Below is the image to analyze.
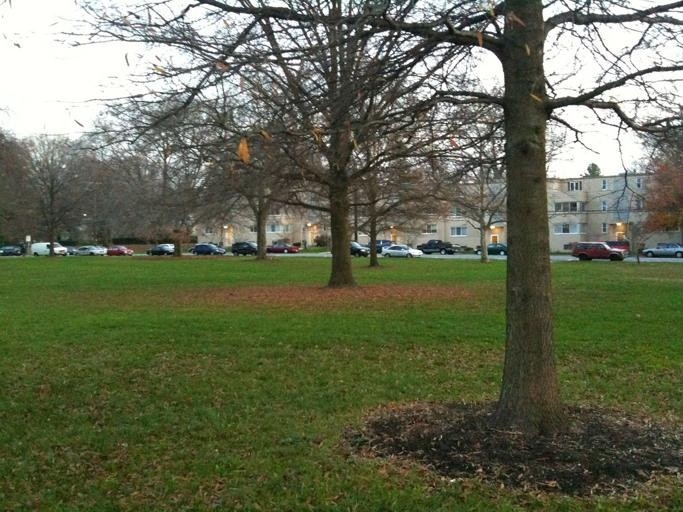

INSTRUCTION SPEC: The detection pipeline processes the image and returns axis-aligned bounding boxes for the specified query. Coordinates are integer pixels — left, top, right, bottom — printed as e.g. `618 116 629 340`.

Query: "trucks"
30 242 67 256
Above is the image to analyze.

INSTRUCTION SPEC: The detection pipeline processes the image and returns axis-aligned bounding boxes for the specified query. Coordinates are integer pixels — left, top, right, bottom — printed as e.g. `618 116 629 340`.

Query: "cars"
146 246 174 256
192 244 225 255
474 243 507 255
330 242 370 258
380 245 423 258
106 246 133 256
642 243 683 258
157 244 174 248
64 245 107 256
188 242 220 252
0 246 23 256
266 243 298 253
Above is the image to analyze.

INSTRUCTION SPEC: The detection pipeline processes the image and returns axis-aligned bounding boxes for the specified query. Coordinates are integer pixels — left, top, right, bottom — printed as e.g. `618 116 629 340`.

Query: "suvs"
231 242 257 256
572 242 625 261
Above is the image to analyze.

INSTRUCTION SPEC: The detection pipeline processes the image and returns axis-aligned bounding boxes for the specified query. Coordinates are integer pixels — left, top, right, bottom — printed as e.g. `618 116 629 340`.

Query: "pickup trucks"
416 240 461 255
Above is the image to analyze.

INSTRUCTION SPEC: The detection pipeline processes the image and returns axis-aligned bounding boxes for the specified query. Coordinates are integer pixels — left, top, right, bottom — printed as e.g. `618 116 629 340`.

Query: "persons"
303 240 306 249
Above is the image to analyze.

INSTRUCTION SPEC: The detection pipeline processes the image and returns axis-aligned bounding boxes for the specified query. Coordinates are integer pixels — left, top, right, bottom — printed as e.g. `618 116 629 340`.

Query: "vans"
368 240 391 253
597 240 629 249
